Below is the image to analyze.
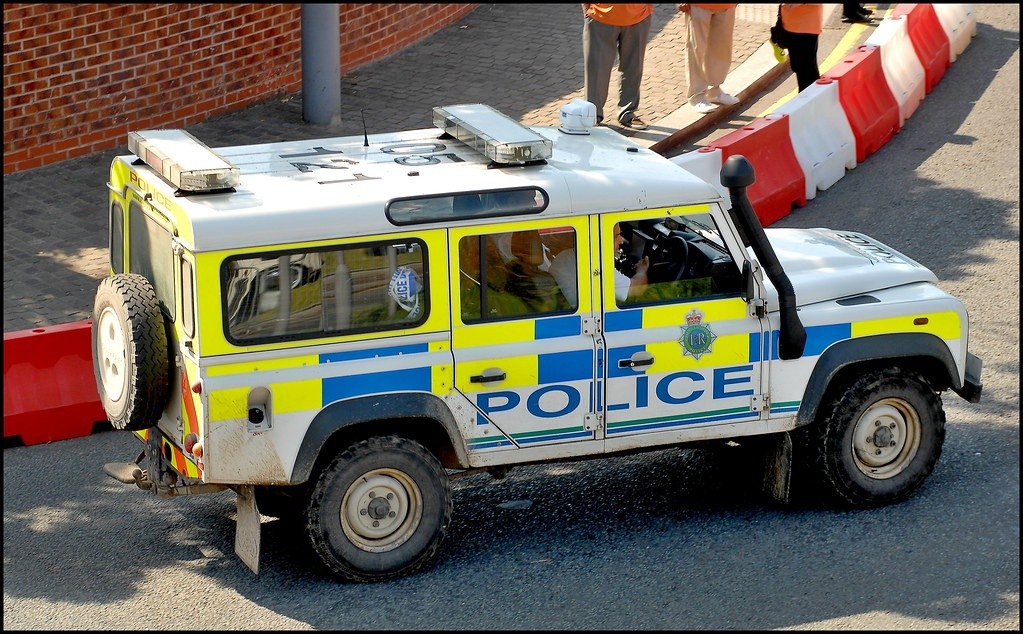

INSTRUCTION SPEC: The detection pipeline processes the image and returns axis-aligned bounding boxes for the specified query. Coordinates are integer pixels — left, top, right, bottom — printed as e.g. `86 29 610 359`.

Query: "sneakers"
769 37 787 64
707 91 740 105
622 117 646 130
692 97 715 114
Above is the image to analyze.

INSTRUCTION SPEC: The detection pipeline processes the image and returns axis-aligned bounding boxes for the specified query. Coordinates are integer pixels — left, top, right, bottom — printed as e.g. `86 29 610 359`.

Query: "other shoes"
842 15 874 23
856 6 873 15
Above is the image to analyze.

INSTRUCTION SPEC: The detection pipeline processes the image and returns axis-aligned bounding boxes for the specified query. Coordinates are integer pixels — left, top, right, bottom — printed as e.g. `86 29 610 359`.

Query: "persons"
774 2 821 93
548 221 653 309
579 1 656 127
677 2 737 115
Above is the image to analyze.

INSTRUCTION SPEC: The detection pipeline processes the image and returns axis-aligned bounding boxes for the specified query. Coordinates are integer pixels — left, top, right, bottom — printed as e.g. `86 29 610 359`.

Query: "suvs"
90 98 983 580
